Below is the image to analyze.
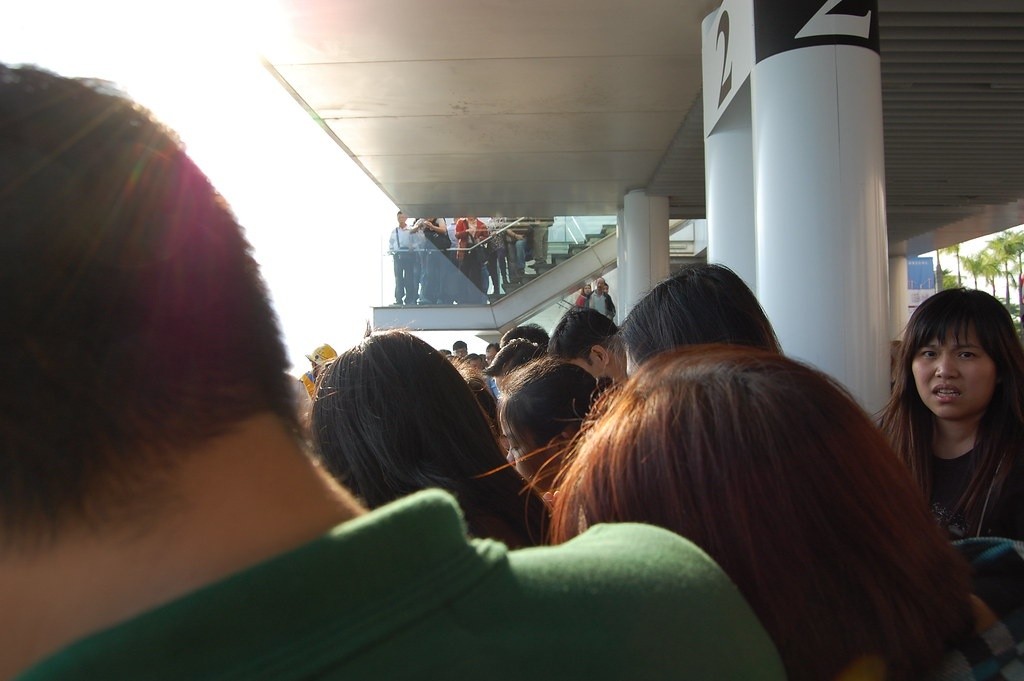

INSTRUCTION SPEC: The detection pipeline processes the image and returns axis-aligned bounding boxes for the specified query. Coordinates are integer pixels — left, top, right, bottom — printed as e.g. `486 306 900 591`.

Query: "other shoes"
528 261 546 268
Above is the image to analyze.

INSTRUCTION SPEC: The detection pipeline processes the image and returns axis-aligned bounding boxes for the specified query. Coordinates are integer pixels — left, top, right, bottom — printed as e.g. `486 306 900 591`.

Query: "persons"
0 74 1024 681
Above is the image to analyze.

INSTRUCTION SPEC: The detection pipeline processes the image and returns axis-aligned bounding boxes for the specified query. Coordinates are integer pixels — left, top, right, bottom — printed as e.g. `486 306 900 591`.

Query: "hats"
305 343 338 367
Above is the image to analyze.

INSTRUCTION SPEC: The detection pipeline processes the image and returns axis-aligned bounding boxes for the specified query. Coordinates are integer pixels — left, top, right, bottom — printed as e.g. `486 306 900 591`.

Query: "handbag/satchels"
464 233 488 269
425 217 451 250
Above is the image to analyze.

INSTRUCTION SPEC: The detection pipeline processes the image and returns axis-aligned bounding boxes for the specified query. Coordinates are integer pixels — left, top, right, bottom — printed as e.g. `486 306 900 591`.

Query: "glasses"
453 350 467 353
